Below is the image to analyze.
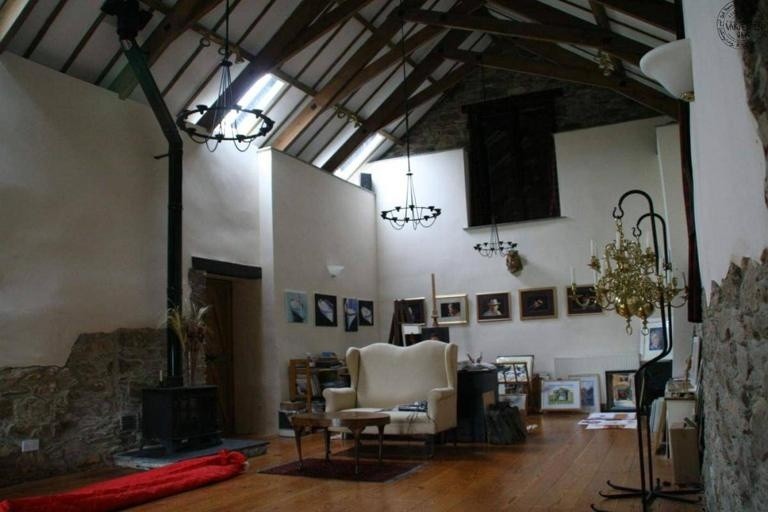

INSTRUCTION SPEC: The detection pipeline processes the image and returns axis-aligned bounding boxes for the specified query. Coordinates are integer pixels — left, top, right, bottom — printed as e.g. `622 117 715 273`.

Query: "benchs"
322 340 459 459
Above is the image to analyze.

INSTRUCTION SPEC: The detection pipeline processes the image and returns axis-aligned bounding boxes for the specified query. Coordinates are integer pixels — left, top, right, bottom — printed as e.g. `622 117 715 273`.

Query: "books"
295 374 321 398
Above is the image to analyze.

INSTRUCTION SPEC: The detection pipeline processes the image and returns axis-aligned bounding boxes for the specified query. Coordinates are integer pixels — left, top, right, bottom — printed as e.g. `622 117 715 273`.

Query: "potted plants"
158 296 217 386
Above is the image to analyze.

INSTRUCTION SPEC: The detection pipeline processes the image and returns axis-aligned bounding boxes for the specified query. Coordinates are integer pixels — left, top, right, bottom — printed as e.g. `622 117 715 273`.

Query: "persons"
446 304 460 316
482 299 502 316
648 329 663 350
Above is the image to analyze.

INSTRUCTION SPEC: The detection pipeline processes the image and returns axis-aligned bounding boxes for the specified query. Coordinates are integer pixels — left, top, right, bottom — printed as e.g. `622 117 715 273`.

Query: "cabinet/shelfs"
140 385 224 456
444 360 507 443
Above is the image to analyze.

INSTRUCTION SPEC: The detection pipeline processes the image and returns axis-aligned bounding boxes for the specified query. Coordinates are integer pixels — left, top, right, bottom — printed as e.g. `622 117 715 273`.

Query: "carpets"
257 457 422 485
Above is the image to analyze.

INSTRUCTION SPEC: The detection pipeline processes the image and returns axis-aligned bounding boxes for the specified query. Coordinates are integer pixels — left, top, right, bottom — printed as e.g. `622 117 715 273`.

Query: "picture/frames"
393 297 426 326
565 284 605 315
476 292 512 322
517 286 558 321
494 317 670 415
434 294 469 326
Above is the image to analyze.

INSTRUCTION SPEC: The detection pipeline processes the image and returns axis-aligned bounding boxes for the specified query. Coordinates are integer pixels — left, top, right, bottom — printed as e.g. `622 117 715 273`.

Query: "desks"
291 410 390 475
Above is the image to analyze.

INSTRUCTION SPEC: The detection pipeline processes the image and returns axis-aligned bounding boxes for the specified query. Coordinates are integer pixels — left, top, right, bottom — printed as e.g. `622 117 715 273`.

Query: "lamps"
326 265 345 278
585 187 688 336
173 0 275 152
472 66 518 259
639 38 693 103
378 0 442 231
